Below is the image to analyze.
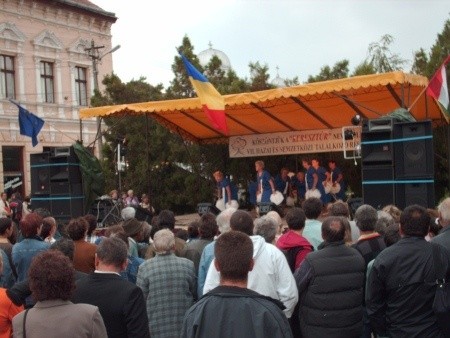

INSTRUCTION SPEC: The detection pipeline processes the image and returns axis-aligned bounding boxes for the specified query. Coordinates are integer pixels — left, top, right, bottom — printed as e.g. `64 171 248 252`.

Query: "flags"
426 58 450 117
181 54 227 134
19 106 44 147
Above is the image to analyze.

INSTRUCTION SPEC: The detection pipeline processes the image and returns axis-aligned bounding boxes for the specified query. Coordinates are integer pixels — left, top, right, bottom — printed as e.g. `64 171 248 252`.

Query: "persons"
239 197 314 272
182 230 294 338
352 204 387 338
301 197 325 251
291 216 365 338
427 197 450 248
328 201 401 276
199 211 234 299
150 210 218 261
12 248 108 338
137 228 198 338
364 205 450 338
0 255 24 338
213 157 343 212
72 237 151 338
202 212 299 318
0 189 155 308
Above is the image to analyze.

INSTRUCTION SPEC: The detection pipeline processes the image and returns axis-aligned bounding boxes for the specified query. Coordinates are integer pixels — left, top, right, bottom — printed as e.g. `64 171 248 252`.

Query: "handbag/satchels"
284 246 304 273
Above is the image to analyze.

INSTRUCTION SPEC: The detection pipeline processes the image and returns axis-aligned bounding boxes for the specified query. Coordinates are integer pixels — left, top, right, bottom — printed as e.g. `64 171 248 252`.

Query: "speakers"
29 146 95 231
361 117 435 211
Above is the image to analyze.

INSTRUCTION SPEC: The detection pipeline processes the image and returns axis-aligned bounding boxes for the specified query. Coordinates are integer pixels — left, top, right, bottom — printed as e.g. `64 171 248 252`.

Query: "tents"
79 70 450 207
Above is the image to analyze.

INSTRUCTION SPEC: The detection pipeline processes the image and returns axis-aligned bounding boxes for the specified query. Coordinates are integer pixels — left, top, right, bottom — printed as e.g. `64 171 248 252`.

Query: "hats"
123 218 143 236
